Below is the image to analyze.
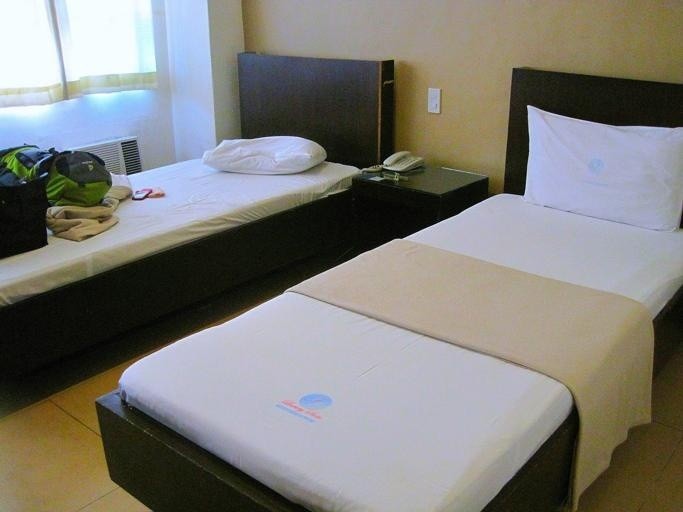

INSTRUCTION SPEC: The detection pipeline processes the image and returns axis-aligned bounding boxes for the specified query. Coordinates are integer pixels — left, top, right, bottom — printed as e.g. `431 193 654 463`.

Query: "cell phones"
132 188 153 200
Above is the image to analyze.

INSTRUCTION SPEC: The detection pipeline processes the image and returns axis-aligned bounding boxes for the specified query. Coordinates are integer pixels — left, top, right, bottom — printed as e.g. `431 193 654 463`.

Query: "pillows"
202 135 326 176
525 105 683 232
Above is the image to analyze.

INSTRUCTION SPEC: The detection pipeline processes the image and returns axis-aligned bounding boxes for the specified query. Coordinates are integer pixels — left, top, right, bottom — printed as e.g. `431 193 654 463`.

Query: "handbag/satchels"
0 162 48 259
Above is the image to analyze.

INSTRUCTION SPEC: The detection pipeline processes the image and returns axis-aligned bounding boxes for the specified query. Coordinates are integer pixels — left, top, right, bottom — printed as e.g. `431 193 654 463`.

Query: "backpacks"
0 143 112 206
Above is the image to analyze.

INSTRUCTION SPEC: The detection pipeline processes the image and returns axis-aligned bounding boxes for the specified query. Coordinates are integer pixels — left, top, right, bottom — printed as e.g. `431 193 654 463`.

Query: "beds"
94 193 683 512
2 156 379 417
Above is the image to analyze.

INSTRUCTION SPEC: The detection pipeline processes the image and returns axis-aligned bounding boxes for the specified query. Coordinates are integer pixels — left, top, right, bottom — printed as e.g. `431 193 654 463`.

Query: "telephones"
382 151 424 174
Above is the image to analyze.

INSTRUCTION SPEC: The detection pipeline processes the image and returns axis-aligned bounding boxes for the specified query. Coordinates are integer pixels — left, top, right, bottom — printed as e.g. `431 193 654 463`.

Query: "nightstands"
353 164 491 248
